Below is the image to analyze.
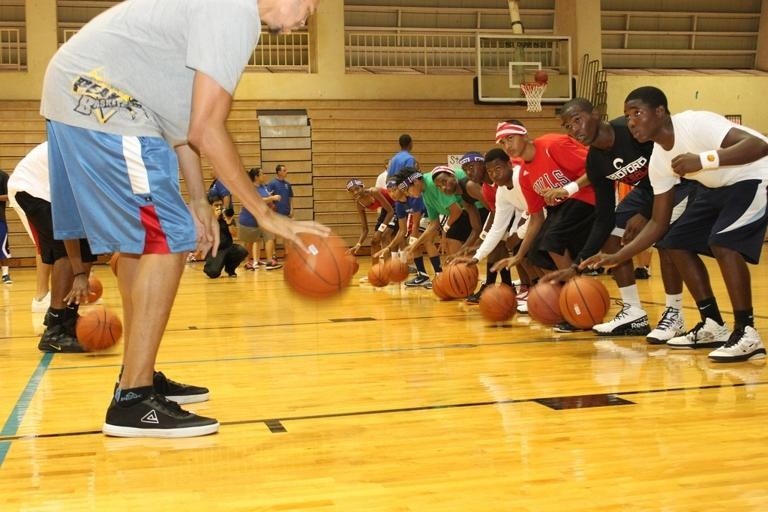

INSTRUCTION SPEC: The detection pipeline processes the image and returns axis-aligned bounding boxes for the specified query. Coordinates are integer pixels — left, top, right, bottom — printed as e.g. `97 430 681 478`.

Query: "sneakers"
1 274 12 284
265 261 282 270
103 395 219 439
646 306 686 344
245 262 254 270
517 301 528 312
553 322 584 332
43 310 80 325
225 269 237 277
359 275 369 283
582 268 598 276
114 372 210 405
253 261 259 269
592 301 651 336
32 292 51 312
667 318 730 348
38 325 82 352
515 287 528 300
259 261 265 265
708 325 766 361
424 279 432 289
634 266 651 279
404 273 430 286
464 284 485 305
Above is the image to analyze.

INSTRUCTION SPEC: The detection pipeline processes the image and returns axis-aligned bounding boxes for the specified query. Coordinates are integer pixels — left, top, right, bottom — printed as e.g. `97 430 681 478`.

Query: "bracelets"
73 271 86 277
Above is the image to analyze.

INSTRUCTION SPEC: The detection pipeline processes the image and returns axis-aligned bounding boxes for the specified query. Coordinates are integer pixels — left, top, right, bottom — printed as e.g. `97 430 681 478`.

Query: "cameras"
217 208 234 221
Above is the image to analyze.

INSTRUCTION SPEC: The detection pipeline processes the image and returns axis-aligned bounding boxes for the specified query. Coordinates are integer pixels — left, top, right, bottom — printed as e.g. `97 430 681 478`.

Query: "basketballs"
385 259 409 282
432 274 454 299
479 284 517 322
351 255 360 274
527 280 565 324
286 227 354 298
369 263 390 286
559 276 610 329
535 70 548 83
80 275 103 305
442 262 478 298
76 307 122 351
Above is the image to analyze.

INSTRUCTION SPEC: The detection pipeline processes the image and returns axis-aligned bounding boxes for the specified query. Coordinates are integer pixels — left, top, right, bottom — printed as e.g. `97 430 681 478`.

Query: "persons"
203 194 249 277
14 196 52 310
238 168 282 270
0 171 13 283
35 0 316 439
267 165 294 258
209 168 233 213
6 138 102 352
347 87 766 363
